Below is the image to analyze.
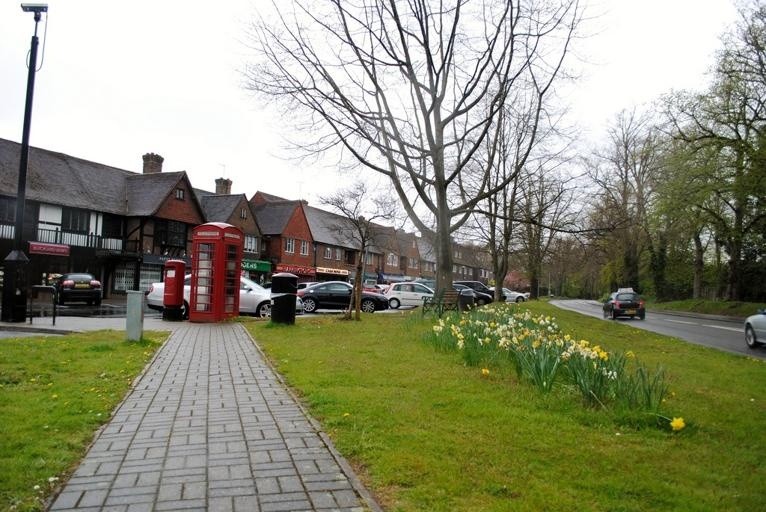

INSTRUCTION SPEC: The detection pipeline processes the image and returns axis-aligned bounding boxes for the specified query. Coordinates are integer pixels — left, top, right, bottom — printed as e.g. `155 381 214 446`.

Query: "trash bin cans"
271 272 299 323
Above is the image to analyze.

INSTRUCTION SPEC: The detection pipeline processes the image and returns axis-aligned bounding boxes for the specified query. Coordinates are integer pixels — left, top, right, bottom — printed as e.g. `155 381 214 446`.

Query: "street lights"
11 2 47 251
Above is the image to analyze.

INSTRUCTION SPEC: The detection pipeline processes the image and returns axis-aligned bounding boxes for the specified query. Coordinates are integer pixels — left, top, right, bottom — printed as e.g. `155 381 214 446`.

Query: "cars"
146 270 304 319
603 287 646 320
298 280 528 314
52 273 103 307
744 308 766 348
